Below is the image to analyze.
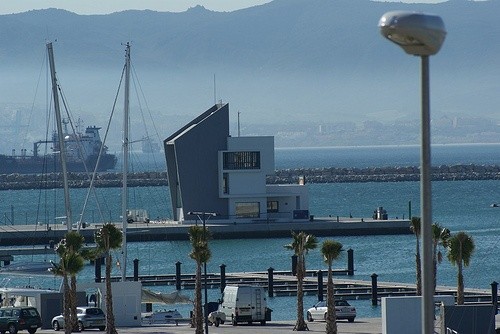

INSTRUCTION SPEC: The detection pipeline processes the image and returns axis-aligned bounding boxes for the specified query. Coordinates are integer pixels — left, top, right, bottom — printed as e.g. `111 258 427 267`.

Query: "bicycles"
154 216 167 224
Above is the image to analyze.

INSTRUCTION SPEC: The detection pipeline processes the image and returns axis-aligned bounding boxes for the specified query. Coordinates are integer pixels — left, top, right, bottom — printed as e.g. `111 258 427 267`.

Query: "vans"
218 284 268 327
127 209 150 223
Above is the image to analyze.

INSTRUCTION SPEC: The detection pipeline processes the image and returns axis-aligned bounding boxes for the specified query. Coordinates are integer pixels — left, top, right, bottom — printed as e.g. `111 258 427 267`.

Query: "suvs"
0 306 44 334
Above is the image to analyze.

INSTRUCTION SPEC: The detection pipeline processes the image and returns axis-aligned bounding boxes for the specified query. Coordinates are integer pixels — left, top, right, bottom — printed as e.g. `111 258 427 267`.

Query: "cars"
307 300 357 322
51 307 107 332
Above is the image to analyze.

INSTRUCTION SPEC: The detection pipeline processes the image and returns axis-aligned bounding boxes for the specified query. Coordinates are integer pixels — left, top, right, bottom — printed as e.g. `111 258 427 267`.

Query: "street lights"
378 10 448 334
187 210 221 334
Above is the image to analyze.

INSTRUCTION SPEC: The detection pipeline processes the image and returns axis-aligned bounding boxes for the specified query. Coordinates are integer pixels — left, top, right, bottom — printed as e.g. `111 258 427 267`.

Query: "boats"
0 117 117 173
